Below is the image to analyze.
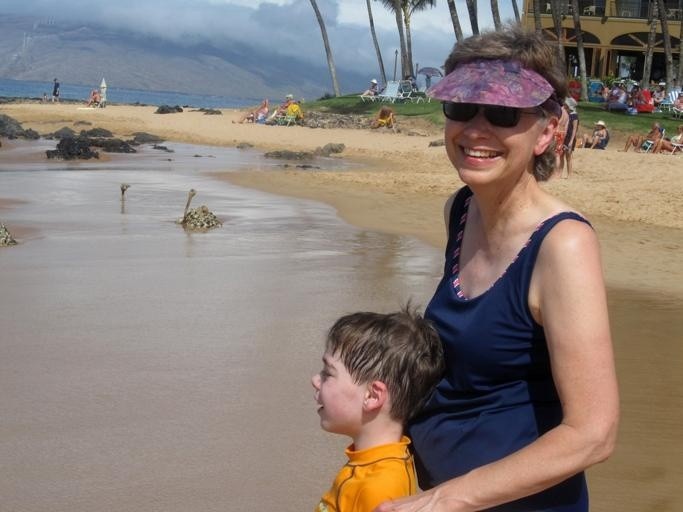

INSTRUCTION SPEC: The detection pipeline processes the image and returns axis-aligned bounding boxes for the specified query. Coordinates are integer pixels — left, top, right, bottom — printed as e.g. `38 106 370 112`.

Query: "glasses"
438 97 548 129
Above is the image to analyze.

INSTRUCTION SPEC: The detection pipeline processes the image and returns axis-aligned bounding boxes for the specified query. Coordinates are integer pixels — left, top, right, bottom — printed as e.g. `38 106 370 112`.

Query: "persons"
361 79 378 97
85 90 100 107
307 303 446 511
374 106 395 128
375 28 624 512
264 94 297 125
51 77 59 104
558 77 683 156
556 96 579 180
231 98 268 127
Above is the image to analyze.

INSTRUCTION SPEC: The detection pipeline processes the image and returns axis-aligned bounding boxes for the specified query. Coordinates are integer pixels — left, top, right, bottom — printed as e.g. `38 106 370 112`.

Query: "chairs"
591 129 609 149
359 79 432 104
637 128 683 155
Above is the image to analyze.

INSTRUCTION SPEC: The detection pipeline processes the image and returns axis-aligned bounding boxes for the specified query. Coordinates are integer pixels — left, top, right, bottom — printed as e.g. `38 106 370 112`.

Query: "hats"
594 120 606 128
370 78 378 85
285 94 294 100
564 96 577 111
424 58 561 111
